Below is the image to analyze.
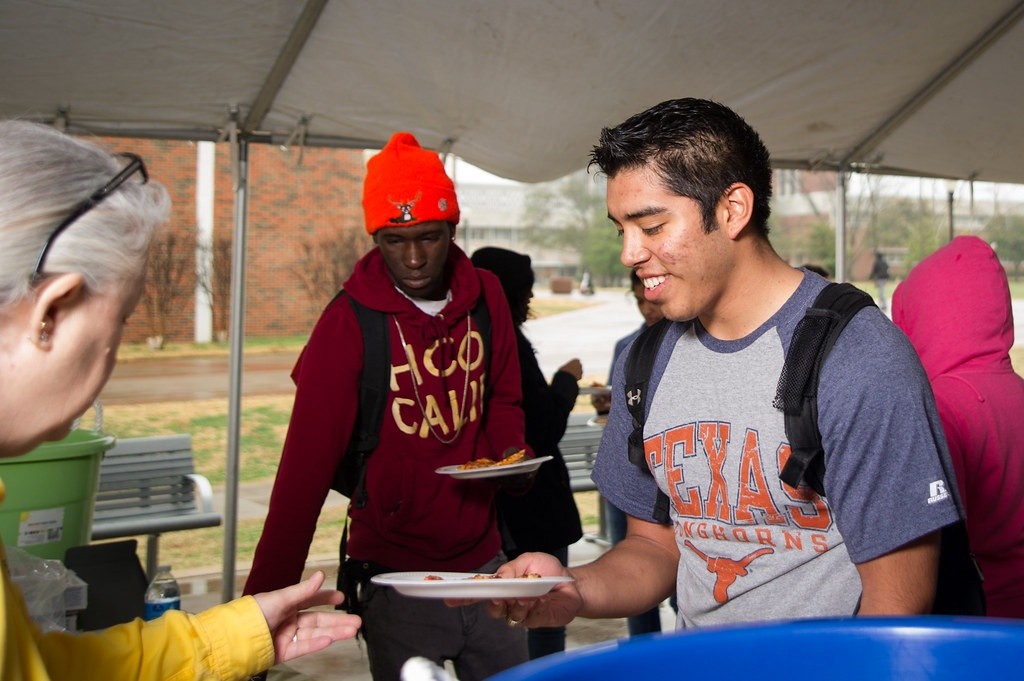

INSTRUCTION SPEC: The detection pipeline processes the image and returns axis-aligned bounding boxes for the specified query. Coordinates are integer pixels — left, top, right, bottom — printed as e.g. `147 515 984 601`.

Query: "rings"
292 627 300 644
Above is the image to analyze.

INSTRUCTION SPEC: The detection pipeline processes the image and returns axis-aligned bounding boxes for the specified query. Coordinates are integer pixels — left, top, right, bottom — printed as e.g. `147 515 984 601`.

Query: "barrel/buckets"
0 428 116 564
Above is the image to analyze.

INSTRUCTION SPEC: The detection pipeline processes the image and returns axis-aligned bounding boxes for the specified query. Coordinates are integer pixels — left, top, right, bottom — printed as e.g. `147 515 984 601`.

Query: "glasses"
29 151 150 307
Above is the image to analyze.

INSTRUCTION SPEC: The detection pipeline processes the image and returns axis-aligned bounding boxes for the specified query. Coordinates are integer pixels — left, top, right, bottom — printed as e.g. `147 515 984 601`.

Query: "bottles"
145 564 180 621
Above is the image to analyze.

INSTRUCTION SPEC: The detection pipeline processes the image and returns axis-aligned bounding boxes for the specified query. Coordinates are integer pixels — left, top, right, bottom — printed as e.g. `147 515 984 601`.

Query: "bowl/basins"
482 615 1024 681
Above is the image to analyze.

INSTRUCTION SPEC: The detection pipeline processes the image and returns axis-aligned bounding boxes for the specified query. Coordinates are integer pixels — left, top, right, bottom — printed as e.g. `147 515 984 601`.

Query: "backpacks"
931 519 988 616
334 557 367 642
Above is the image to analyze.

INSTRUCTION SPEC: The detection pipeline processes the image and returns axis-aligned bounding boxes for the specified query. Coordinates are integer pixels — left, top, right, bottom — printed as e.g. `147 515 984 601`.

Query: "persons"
240 98 1024 681
0 119 362 681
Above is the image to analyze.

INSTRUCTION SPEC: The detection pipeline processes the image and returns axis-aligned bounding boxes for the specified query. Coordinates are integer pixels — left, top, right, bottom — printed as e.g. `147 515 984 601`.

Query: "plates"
587 416 610 427
371 572 573 598
434 455 554 479
579 385 612 394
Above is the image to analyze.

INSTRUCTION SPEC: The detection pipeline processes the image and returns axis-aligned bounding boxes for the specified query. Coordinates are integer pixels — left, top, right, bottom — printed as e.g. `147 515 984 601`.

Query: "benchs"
91 433 222 586
557 412 608 542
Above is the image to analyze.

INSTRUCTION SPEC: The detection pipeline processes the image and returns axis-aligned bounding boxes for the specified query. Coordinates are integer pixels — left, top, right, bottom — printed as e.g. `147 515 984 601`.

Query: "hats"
361 131 461 235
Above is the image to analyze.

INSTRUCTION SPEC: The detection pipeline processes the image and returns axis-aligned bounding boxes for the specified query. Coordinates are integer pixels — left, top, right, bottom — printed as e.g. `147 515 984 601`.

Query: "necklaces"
390 312 472 443
